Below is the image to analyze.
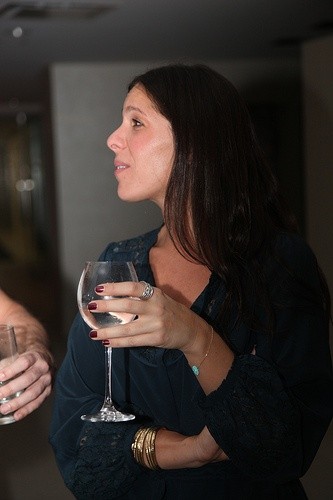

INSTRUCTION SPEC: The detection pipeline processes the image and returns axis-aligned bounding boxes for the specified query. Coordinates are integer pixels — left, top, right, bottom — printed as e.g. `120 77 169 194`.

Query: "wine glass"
77 262 139 422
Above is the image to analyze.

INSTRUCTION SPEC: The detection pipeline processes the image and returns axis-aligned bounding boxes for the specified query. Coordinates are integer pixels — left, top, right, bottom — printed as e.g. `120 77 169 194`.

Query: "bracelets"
132 428 162 472
191 327 214 375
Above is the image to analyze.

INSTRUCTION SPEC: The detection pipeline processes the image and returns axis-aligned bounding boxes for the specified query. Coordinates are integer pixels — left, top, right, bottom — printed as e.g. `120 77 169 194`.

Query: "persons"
50 62 333 500
0 286 53 426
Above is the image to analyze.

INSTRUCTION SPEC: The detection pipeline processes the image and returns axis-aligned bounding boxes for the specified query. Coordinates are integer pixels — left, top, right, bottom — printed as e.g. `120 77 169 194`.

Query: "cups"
0 324 25 425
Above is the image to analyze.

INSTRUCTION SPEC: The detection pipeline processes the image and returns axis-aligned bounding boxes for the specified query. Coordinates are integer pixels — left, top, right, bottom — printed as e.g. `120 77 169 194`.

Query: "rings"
141 282 154 301
